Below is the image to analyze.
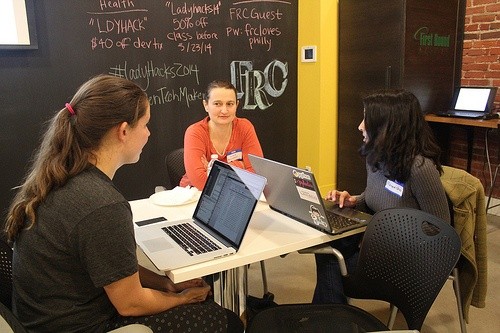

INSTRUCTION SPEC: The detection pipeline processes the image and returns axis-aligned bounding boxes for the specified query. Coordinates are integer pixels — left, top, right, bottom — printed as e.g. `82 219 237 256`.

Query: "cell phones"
135 217 167 227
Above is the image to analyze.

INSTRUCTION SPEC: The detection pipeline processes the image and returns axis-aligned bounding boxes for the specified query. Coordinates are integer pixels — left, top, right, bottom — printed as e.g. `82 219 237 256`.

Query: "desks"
425 114 500 174
128 189 367 333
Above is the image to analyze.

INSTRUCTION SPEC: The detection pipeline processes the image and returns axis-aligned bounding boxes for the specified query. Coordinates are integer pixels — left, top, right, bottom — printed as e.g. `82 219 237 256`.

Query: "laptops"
247 153 373 236
133 159 267 272
434 86 498 119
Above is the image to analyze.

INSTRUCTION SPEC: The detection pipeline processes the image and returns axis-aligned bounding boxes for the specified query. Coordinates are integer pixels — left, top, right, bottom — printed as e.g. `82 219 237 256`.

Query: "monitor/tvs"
0 0 38 50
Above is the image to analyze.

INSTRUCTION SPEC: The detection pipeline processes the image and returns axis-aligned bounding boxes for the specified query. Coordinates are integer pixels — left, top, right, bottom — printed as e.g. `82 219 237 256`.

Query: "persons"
5 74 245 333
179 80 264 282
310 89 451 305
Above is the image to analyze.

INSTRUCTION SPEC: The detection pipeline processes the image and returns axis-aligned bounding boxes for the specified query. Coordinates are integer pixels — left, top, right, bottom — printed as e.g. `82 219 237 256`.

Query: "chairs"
247 207 463 333
387 165 485 333
164 148 269 296
